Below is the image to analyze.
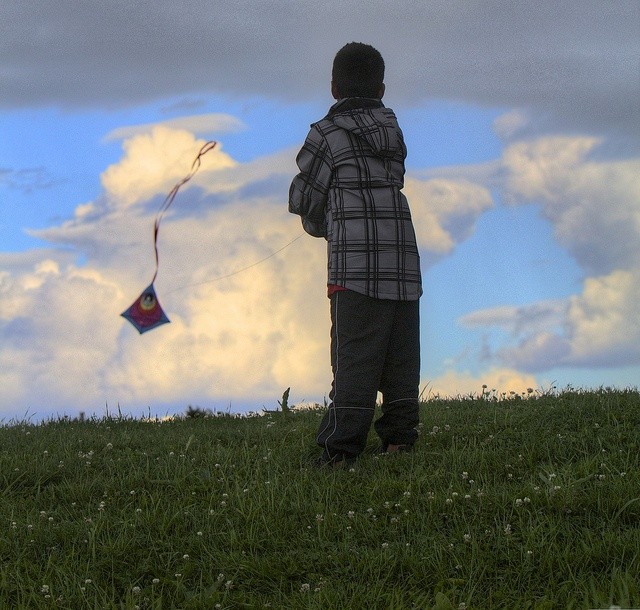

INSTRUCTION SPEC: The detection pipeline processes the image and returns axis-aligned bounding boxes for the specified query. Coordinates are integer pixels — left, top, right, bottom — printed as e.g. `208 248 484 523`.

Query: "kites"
116 140 217 337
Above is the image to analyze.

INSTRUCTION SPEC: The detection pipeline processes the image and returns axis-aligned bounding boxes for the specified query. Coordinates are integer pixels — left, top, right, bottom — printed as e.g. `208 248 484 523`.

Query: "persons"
289 41 422 461
